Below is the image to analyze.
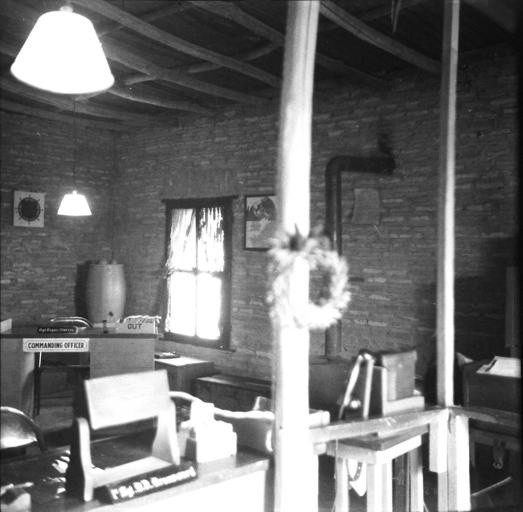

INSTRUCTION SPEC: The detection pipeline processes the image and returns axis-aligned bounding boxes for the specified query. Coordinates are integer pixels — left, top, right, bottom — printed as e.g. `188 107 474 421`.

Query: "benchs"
190 371 271 413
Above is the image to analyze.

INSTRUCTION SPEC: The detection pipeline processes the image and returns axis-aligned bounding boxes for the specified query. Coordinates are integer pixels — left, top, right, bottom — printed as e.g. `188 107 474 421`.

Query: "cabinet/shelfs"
154 354 213 407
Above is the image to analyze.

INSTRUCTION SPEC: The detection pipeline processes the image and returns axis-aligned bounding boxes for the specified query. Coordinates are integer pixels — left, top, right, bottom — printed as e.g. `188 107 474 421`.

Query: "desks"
1 423 269 509
1 322 157 420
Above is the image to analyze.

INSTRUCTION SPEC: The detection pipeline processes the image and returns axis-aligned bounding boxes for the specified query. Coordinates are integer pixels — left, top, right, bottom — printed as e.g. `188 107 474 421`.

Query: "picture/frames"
242 193 277 252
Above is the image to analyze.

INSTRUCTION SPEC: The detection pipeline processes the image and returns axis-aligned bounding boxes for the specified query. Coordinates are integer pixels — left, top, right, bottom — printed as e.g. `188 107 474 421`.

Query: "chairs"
31 316 94 420
322 347 428 511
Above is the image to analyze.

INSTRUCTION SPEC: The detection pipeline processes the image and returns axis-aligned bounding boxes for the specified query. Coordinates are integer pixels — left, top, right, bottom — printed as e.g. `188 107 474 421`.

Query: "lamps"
8 3 116 218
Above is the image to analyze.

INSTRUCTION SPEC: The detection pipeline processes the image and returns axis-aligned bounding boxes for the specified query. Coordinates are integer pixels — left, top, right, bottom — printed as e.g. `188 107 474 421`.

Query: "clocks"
11 189 48 231
